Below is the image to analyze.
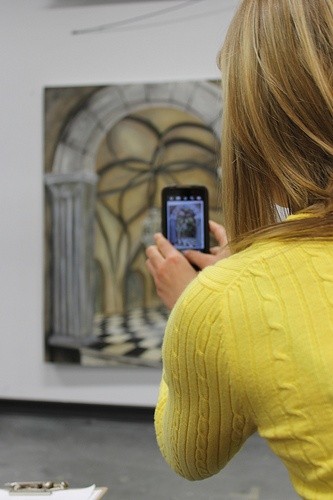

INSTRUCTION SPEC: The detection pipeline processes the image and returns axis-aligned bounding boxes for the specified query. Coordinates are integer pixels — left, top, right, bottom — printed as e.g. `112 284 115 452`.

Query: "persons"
136 1 333 500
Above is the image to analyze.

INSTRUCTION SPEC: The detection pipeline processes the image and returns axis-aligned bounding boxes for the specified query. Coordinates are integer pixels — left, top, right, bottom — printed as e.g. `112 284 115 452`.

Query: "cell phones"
161 185 210 271
